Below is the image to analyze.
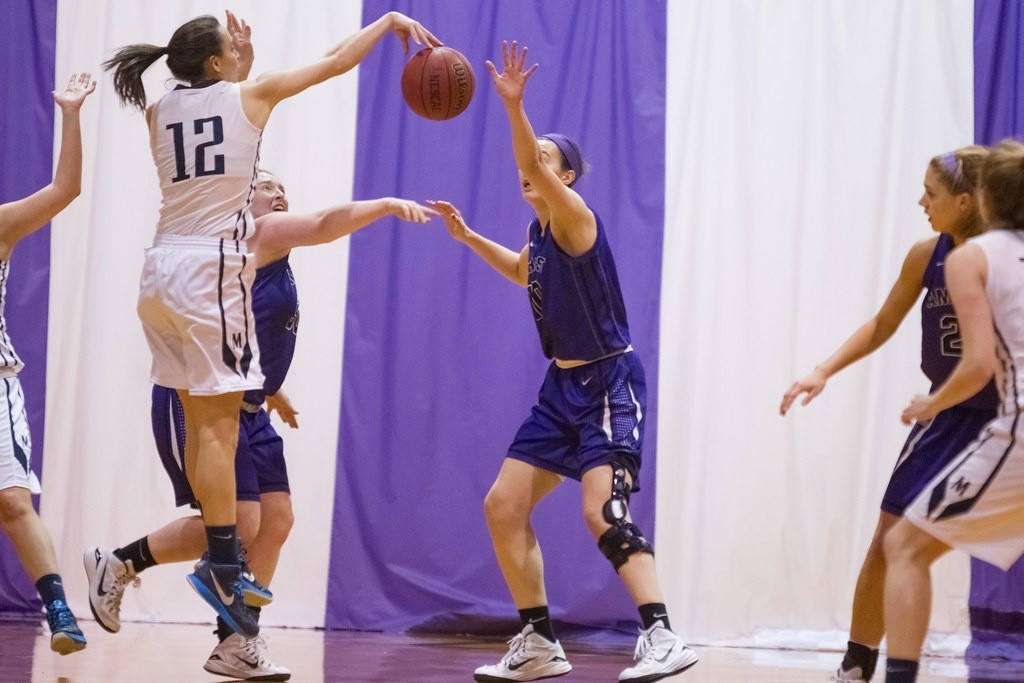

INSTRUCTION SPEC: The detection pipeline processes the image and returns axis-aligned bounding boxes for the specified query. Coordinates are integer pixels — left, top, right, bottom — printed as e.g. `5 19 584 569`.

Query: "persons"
424 35 699 683
778 136 1024 683
80 8 440 683
0 70 98 656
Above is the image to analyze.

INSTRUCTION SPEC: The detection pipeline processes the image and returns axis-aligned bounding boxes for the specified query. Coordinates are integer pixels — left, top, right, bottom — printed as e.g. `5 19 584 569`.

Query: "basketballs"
399 45 477 123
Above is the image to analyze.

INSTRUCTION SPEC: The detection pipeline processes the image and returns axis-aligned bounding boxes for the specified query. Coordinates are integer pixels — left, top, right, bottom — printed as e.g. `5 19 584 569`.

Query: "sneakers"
828 660 866 683
203 632 291 681
618 620 700 682
474 623 572 682
83 546 141 633
194 537 273 608
186 561 260 638
45 600 88 656
